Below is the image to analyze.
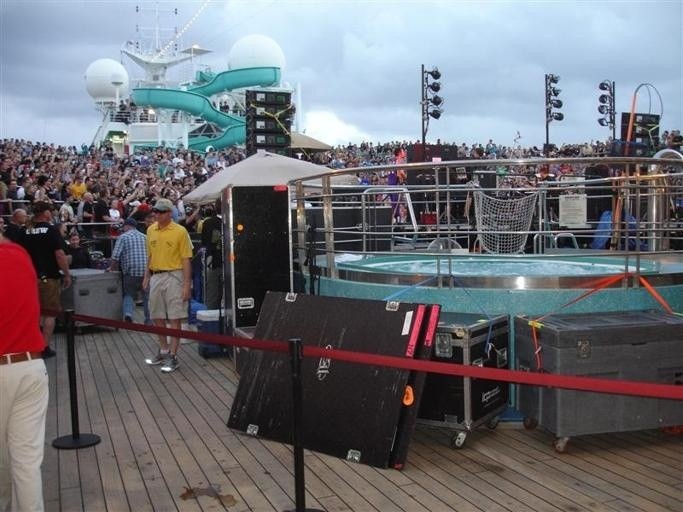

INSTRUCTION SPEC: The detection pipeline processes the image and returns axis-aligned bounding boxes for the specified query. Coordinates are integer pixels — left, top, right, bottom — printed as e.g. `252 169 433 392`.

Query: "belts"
0 352 43 363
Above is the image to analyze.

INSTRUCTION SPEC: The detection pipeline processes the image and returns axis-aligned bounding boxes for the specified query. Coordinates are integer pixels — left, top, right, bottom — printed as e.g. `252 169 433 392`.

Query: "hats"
33 201 55 212
122 218 138 226
152 198 173 212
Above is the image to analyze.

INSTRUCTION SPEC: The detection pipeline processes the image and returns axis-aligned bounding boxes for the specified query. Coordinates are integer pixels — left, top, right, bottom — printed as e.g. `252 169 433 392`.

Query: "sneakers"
145 347 171 364
42 345 55 358
160 355 180 372
124 311 133 322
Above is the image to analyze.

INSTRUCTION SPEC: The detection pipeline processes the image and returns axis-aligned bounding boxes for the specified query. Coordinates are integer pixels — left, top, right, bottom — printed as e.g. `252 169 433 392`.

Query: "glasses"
152 209 166 214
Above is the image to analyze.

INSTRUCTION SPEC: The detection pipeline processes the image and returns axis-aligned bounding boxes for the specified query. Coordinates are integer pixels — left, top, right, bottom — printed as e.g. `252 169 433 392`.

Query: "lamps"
599 83 614 127
427 69 441 118
546 73 564 122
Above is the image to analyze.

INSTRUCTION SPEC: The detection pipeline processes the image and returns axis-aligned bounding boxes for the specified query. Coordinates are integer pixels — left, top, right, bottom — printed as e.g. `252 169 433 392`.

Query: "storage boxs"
514 309 683 438
417 310 510 427
195 308 222 355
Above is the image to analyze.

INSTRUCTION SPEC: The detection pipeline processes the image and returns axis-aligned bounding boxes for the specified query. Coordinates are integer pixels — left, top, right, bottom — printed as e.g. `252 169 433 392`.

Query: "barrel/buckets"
195 309 219 357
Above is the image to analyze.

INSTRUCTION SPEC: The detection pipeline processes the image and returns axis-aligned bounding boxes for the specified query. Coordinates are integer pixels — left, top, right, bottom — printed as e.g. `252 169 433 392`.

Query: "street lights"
544 73 563 157
598 79 616 157
422 64 442 162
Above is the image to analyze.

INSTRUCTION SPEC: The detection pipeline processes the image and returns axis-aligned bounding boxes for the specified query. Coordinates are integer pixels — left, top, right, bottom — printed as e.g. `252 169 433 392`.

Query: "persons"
199 209 222 310
143 199 194 372
106 218 152 326
15 202 71 359
2 236 49 512
0 99 682 270
